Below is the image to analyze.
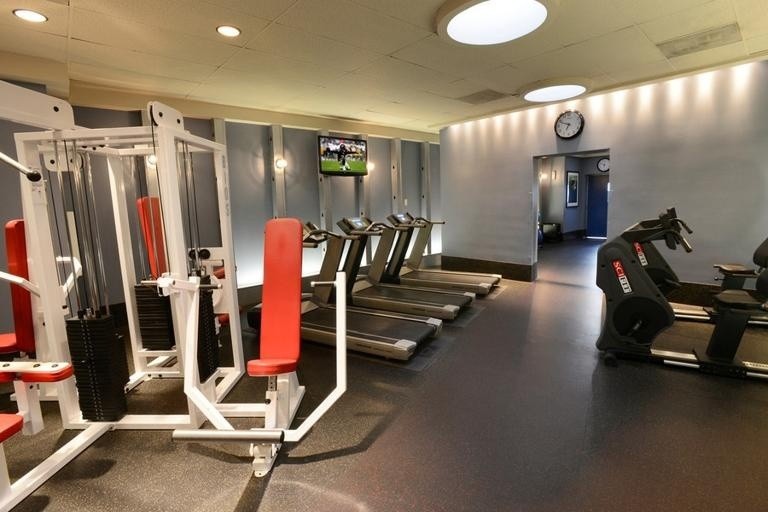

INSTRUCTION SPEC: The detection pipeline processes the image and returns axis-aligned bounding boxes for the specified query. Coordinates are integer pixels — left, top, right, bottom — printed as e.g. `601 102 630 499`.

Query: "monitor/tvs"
318 134 368 176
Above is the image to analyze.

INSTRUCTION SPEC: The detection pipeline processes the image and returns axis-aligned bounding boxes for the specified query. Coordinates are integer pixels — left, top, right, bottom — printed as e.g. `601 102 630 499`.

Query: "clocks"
554 110 584 140
597 158 609 172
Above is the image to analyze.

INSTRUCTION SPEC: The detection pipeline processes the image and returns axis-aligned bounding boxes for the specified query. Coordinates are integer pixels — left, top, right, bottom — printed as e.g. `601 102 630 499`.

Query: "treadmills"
247 223 442 360
384 213 502 295
329 218 475 322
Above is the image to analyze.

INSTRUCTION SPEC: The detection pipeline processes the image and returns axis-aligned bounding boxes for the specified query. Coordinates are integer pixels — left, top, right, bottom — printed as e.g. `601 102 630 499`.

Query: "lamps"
276 159 290 169
517 76 591 105
432 0 552 47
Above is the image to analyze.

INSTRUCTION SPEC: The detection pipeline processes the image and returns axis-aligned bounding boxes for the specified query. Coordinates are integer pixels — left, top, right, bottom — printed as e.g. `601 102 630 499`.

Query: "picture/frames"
566 171 579 208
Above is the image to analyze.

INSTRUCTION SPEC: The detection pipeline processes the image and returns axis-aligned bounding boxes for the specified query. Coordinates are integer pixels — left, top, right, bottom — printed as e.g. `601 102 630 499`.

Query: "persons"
570 179 577 192
320 137 365 172
